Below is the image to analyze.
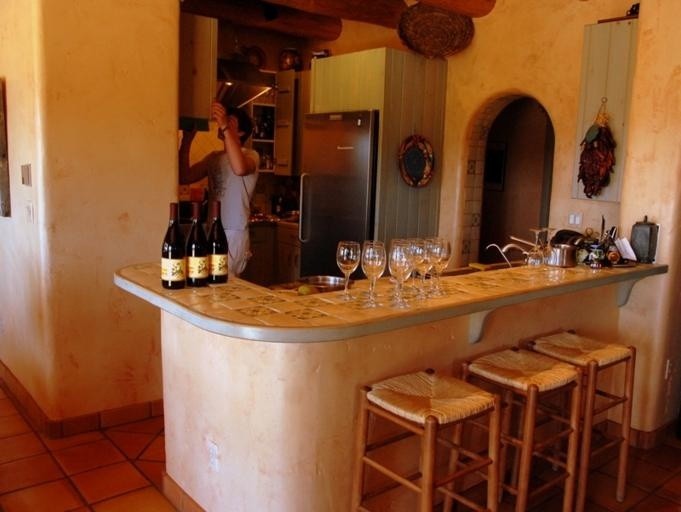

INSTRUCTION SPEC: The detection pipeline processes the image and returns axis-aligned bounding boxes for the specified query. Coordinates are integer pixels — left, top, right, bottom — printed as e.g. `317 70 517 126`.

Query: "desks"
113 247 668 511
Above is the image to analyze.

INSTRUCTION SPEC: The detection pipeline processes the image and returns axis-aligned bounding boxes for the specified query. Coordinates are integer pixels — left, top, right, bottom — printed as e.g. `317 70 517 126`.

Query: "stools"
511 329 638 511
351 369 503 511
443 342 587 512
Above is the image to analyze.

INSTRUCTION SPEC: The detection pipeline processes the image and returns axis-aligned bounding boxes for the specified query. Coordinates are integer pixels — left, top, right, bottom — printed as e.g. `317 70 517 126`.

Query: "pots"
510 234 580 269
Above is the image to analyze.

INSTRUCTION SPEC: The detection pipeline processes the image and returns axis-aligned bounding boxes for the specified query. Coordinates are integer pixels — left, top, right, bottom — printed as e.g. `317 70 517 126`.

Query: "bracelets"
219 125 231 134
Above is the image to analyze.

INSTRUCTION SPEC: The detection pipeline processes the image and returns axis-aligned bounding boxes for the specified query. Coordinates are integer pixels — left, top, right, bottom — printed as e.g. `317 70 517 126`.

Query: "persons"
177 103 261 278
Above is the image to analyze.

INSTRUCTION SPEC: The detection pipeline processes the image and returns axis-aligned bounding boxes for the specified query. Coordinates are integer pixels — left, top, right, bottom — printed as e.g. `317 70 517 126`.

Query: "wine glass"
526 225 558 270
335 237 453 308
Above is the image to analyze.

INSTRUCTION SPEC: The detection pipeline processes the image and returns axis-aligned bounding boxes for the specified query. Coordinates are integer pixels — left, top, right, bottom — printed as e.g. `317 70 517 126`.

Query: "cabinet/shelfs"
275 219 301 283
181 13 218 122
275 70 311 177
215 69 275 173
241 221 276 287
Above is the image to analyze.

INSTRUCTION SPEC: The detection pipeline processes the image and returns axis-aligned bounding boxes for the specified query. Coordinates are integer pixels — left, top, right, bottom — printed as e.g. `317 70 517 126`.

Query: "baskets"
397 0 476 58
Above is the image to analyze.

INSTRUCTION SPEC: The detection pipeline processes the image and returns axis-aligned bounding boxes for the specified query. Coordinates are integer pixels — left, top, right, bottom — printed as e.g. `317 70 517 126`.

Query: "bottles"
160 200 230 290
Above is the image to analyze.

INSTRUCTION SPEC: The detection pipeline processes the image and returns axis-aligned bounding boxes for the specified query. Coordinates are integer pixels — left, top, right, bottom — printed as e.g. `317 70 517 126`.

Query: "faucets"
502 243 527 256
485 244 513 268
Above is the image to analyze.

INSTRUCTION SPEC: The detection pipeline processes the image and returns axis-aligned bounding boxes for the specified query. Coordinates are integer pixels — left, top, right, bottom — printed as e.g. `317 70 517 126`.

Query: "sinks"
490 262 525 268
444 268 479 276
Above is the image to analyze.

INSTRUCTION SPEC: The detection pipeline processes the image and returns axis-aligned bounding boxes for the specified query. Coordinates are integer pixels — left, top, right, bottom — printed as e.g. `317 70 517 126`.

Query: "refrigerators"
298 112 379 277
